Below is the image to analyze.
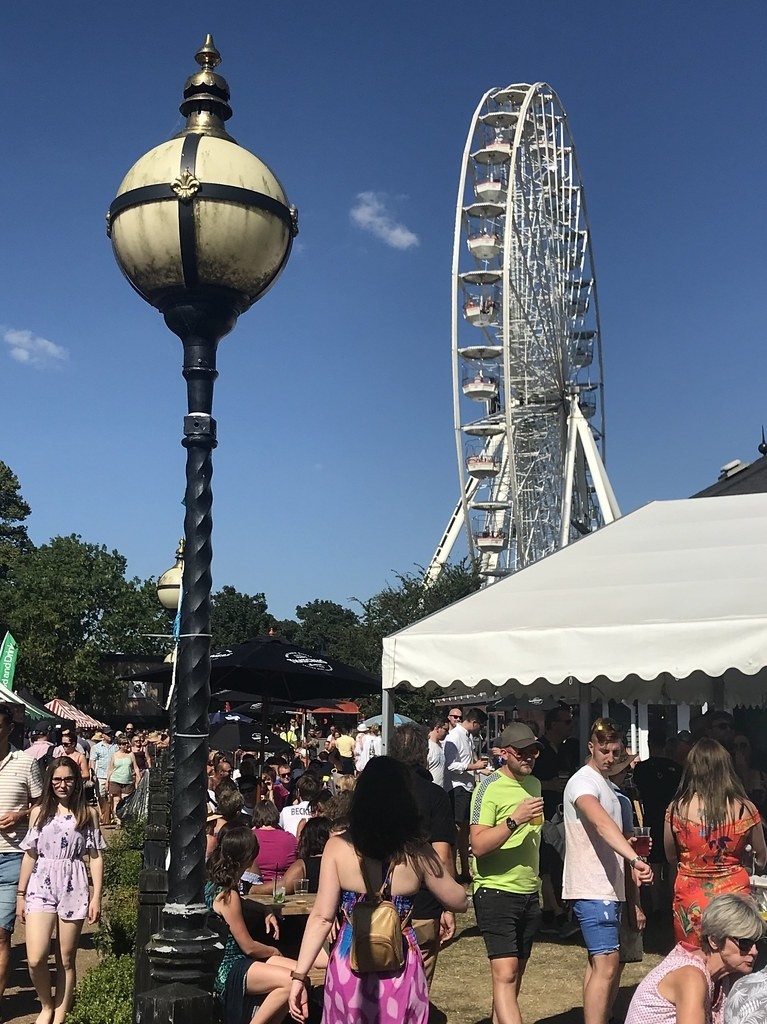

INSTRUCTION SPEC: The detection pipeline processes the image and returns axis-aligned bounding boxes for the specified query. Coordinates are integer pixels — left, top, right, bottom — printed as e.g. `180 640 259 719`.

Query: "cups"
481 757 489 767
273 877 287 904
633 826 651 856
527 796 542 826
293 878 309 905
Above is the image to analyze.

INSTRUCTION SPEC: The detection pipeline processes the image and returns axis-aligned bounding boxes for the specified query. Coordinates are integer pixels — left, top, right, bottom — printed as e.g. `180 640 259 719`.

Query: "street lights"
100 31 304 1024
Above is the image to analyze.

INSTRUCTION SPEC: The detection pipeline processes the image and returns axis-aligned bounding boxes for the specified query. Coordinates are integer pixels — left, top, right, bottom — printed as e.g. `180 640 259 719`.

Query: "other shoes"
98 816 122 830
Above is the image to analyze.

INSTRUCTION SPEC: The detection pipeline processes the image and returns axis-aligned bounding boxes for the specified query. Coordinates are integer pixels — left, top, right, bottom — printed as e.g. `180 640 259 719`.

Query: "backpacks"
329 830 414 976
37 744 56 782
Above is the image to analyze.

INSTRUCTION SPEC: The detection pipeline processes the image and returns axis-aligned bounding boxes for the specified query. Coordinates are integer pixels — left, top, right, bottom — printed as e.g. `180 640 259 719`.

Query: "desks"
239 892 317 917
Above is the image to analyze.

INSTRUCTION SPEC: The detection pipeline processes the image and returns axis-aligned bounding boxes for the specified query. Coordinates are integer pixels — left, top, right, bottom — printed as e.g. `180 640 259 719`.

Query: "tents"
1 681 111 730
379 490 767 761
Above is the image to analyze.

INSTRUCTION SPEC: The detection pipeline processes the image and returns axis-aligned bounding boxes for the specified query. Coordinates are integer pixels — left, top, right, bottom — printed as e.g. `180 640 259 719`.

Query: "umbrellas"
117 629 420 770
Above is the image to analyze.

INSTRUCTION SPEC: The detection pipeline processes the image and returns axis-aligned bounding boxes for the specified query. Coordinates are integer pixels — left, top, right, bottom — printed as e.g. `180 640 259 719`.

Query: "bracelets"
16 889 25 893
288 970 308 982
16 894 25 896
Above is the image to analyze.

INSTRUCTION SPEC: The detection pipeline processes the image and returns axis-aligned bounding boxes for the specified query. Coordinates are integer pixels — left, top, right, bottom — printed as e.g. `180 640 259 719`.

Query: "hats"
206 802 223 823
356 723 370 731
500 722 544 753
34 720 58 734
92 726 123 741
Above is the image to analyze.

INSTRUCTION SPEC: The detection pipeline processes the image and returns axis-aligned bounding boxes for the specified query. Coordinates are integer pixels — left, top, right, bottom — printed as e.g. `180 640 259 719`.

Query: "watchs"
506 817 517 831
628 856 644 869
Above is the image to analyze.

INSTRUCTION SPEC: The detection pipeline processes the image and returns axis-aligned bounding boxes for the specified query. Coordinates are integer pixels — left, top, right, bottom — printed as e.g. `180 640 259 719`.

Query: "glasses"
121 742 129 746
59 741 74 747
556 719 572 725
590 721 623 742
449 715 462 720
134 740 141 743
225 769 231 773
51 776 76 789
505 748 540 761
732 742 749 748
336 785 341 789
280 772 291 778
239 786 257 794
710 722 737 730
731 936 767 953
126 728 133 730
476 721 484 729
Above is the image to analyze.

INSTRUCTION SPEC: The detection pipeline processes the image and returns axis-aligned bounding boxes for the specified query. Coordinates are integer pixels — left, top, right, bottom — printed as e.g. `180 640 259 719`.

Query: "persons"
0 708 45 1010
665 738 767 949
22 707 767 1024
14 754 108 1024
